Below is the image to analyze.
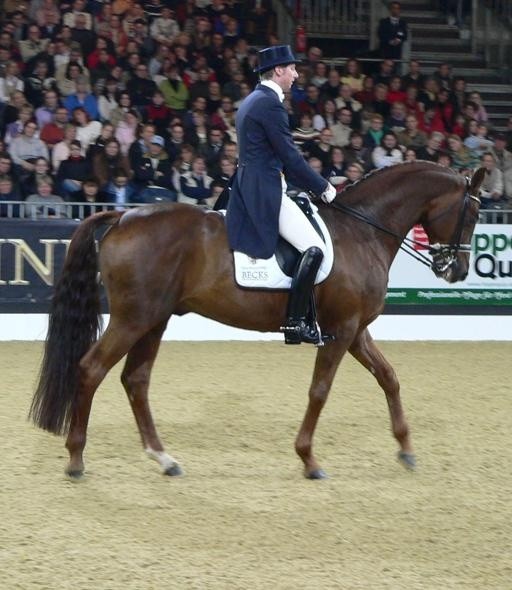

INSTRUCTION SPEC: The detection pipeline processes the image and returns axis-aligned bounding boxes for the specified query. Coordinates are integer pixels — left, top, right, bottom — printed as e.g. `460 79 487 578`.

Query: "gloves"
320 181 337 204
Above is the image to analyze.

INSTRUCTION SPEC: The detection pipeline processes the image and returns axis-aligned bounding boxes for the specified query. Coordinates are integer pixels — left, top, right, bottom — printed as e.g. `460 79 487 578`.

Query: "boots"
279 246 335 345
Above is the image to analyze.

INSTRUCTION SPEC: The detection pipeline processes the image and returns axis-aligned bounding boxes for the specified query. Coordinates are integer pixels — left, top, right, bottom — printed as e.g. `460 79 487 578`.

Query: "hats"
253 45 301 74
150 136 165 147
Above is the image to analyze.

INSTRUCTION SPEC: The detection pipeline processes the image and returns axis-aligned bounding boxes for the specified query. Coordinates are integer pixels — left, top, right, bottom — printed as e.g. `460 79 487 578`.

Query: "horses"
25 158 490 485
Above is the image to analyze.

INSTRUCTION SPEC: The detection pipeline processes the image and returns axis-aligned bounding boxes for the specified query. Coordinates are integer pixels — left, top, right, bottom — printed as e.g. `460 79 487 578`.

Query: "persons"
226 45 337 345
281 40 512 222
2 1 263 214
375 2 409 60
